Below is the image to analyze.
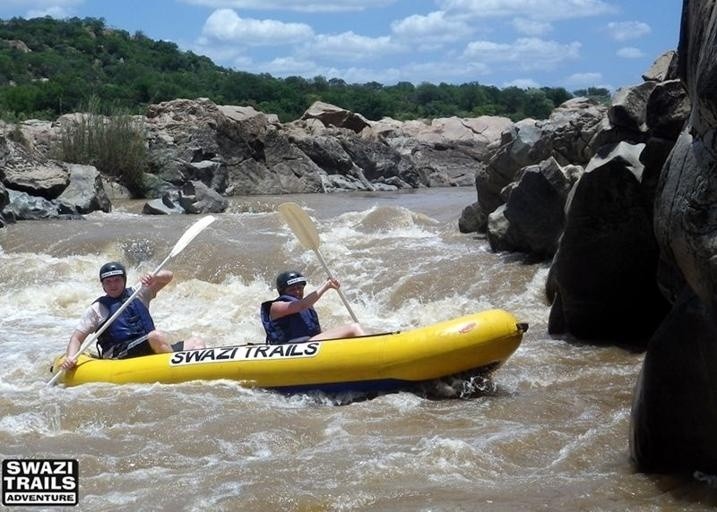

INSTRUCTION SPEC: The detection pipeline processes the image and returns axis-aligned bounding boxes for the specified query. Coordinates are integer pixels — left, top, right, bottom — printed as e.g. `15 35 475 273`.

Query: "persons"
58 261 205 371
259 269 362 346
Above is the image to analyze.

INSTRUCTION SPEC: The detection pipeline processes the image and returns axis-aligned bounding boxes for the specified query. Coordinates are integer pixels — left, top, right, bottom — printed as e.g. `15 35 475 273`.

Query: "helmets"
276 271 306 292
100 262 126 282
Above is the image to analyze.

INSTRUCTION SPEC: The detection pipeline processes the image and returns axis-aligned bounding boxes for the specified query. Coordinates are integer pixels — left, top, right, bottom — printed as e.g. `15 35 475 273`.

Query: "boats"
50 309 529 401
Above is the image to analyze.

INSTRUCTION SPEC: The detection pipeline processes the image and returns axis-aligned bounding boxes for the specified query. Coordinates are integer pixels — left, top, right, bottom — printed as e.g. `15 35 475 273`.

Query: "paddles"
278 203 358 324
44 213 215 391
